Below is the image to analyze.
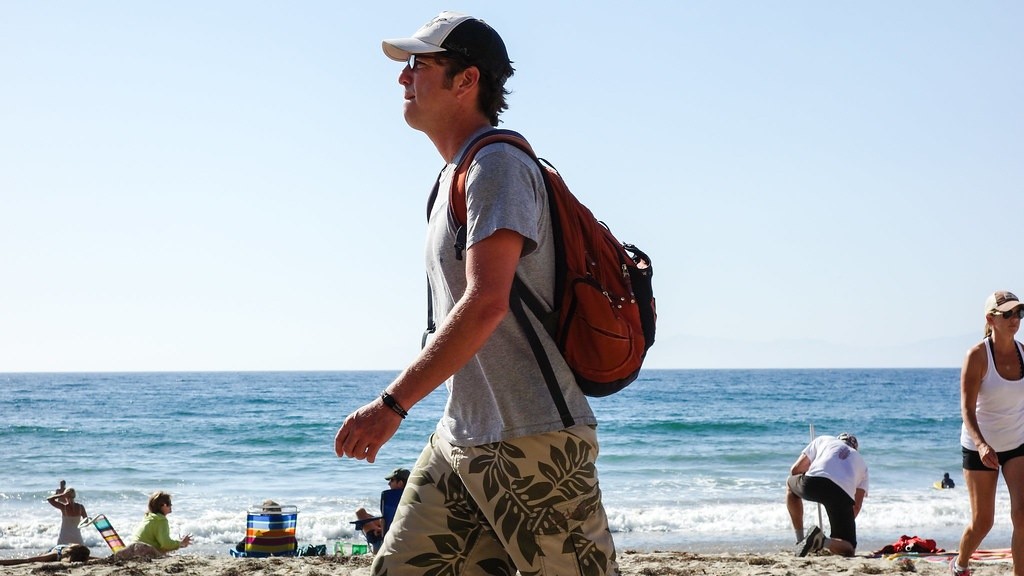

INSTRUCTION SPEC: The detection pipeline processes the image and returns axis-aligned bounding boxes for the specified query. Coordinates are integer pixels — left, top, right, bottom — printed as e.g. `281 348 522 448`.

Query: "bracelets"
378 390 408 419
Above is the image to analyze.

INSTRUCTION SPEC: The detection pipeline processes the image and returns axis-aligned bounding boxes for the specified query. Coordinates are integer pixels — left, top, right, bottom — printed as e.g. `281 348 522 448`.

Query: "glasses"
161 502 171 507
993 308 1024 319
407 54 470 69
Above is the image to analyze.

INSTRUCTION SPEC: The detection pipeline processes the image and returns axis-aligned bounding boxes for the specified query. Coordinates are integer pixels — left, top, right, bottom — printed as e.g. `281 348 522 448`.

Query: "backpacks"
426 130 657 397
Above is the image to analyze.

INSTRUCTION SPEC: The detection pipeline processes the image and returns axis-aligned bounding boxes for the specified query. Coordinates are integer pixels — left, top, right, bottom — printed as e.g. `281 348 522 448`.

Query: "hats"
985 289 1024 312
382 11 509 84
837 433 858 450
385 468 410 480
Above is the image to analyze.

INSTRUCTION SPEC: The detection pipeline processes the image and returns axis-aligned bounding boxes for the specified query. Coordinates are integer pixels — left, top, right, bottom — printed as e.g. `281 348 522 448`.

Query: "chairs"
77 512 126 553
230 505 303 558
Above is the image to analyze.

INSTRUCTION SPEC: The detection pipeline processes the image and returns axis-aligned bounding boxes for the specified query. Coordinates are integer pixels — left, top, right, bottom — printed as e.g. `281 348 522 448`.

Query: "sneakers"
796 525 826 557
949 555 972 576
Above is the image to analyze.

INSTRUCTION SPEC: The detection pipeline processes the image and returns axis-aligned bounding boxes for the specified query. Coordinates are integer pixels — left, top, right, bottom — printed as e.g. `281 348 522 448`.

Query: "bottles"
334 537 341 557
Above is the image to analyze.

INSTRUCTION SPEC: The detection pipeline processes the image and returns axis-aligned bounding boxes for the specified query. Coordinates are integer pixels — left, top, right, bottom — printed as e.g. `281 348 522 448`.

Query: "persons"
356 468 411 550
56 480 67 503
131 491 194 556
235 499 297 552
334 9 620 576
949 290 1024 576
47 488 88 545
0 543 100 566
787 432 868 557
942 472 954 488
